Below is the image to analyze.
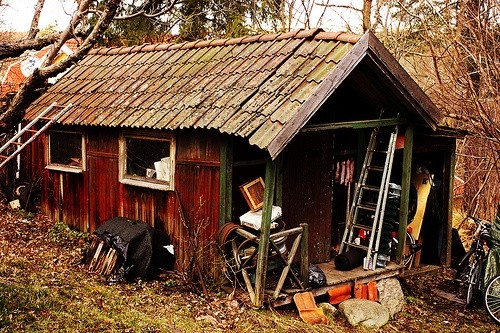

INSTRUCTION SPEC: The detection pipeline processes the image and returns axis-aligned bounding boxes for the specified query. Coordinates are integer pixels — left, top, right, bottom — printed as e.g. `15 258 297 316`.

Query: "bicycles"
483 238 500 325
459 215 492 307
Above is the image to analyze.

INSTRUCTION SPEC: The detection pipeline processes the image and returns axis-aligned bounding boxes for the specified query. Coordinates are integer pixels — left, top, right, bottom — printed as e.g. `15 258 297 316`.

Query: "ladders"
336 104 403 269
0 101 73 168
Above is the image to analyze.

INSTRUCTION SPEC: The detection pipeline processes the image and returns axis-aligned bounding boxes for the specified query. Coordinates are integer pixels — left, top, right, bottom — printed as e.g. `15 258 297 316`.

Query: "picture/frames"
239 176 265 212
